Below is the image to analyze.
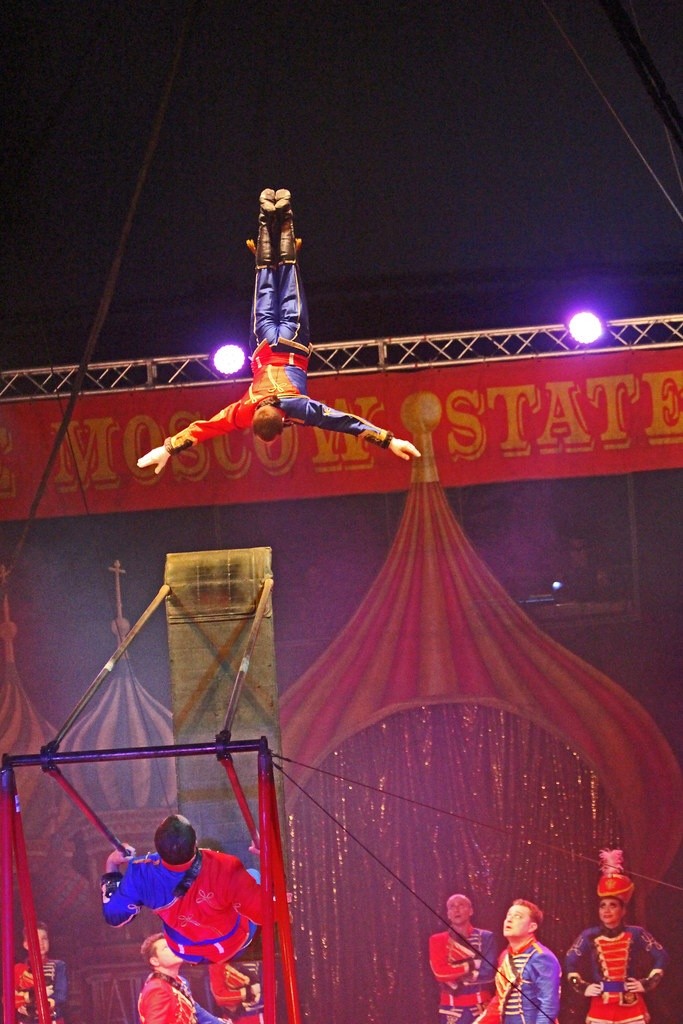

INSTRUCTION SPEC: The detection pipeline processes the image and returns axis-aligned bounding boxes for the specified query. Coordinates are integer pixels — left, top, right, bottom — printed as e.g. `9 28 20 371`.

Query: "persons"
99 814 271 965
137 933 226 1024
15 921 67 1024
207 959 266 1023
137 189 421 475
496 898 563 1024
428 894 500 1024
564 894 670 1024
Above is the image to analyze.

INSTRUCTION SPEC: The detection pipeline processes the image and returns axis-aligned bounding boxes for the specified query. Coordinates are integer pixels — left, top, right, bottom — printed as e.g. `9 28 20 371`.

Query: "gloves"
136 445 171 474
584 983 602 997
626 978 645 993
387 437 421 461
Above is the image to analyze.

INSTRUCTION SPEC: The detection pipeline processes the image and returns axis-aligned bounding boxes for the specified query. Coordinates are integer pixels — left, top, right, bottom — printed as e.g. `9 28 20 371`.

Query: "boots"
274 189 298 267
254 189 278 271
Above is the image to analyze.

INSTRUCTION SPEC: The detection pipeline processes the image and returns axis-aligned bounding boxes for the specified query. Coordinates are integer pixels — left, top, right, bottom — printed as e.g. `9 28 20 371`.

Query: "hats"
597 849 635 908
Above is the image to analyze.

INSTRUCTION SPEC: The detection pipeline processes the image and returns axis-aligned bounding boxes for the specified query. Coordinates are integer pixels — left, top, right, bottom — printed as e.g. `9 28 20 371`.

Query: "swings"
49 545 281 961
